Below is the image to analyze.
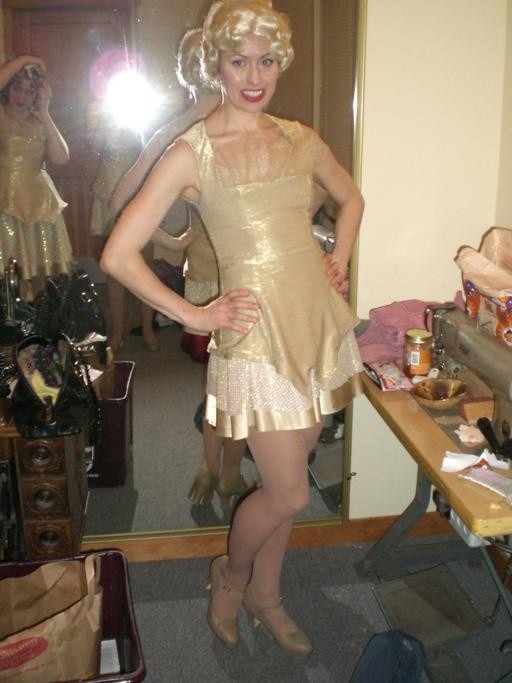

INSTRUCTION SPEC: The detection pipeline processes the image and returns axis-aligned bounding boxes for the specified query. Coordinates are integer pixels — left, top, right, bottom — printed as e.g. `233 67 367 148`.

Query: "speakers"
14 429 91 564
1 432 27 563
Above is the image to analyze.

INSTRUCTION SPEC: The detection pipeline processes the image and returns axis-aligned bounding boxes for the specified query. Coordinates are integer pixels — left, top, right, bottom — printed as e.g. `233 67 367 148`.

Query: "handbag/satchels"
456 227 511 348
44 273 105 342
0 555 104 683
15 333 95 437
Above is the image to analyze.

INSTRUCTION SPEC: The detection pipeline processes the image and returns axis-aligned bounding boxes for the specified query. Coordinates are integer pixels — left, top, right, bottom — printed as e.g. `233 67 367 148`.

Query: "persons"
83 97 161 356
107 27 258 514
98 0 368 659
0 54 75 309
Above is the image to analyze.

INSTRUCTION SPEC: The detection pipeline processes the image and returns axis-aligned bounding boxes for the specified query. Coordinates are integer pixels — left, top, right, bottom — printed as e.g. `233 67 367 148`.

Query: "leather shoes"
130 320 177 335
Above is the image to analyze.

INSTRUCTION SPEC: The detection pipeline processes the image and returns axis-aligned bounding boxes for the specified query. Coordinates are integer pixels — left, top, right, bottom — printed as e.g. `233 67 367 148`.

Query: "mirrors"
0 0 369 545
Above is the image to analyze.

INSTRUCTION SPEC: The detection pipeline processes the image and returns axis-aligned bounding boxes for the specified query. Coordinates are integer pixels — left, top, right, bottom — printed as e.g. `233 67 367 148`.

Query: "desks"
355 362 509 681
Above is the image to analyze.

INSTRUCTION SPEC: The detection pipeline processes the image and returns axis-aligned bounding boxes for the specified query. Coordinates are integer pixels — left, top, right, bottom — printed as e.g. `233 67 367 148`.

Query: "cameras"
26 61 48 87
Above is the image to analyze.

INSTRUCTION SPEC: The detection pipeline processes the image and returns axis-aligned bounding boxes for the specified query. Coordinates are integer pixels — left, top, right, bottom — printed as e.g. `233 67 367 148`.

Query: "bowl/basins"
411 378 469 411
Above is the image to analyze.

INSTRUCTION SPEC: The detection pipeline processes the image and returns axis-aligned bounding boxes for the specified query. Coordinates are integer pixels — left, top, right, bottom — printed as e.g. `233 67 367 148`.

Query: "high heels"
244 585 315 656
202 555 243 645
217 475 256 508
187 468 215 506
